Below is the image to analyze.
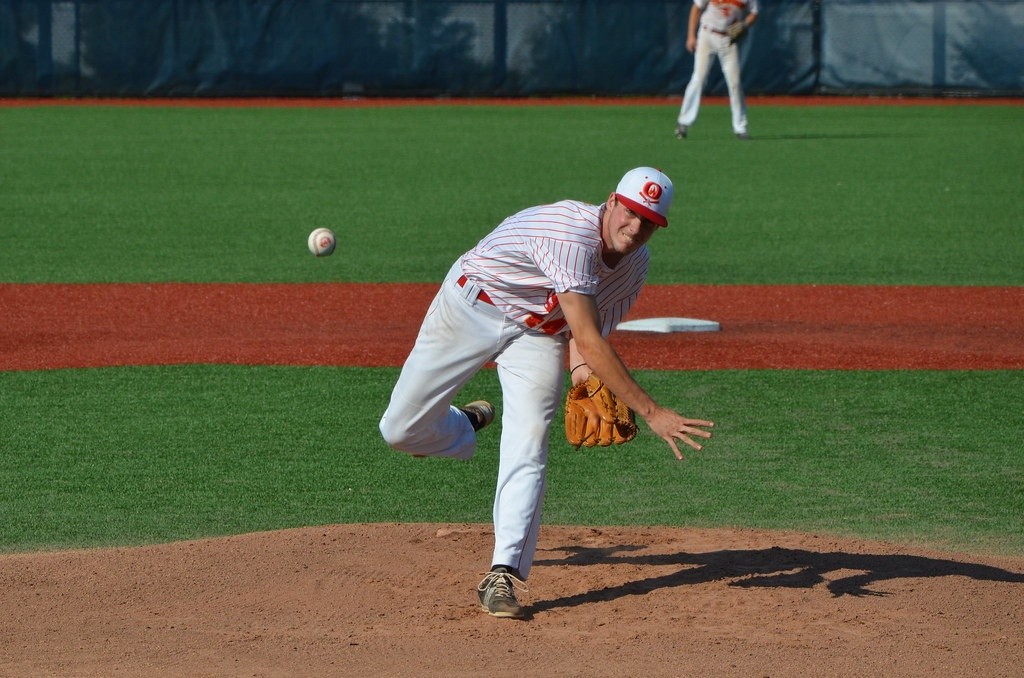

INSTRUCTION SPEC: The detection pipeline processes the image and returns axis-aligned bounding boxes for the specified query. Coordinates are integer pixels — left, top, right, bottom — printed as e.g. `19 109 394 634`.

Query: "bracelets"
570 362 586 375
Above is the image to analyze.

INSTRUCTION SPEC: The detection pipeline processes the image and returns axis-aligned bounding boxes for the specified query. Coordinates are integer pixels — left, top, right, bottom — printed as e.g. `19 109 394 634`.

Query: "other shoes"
675 124 687 139
737 133 751 139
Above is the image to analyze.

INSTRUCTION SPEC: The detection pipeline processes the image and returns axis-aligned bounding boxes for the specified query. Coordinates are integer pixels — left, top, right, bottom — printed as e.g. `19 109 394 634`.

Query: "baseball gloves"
564 371 640 449
725 21 750 45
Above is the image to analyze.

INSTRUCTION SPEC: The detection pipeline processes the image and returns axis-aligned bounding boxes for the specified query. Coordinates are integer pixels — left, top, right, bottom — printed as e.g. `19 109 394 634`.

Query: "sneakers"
478 571 530 618
414 401 494 457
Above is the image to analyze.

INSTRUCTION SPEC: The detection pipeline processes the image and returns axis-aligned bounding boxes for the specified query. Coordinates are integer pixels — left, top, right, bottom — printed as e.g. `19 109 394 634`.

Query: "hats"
615 167 673 227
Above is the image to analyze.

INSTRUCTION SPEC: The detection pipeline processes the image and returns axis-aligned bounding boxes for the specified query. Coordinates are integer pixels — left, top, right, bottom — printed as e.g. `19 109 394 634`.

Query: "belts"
457 274 495 305
704 25 728 36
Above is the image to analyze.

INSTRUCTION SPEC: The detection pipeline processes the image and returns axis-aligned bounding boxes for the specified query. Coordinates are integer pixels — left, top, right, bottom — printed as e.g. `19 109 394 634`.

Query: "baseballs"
307 227 337 257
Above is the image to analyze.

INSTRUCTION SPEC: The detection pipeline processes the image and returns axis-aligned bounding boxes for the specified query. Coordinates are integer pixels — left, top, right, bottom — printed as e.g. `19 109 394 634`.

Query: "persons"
378 166 715 620
674 0 759 140
